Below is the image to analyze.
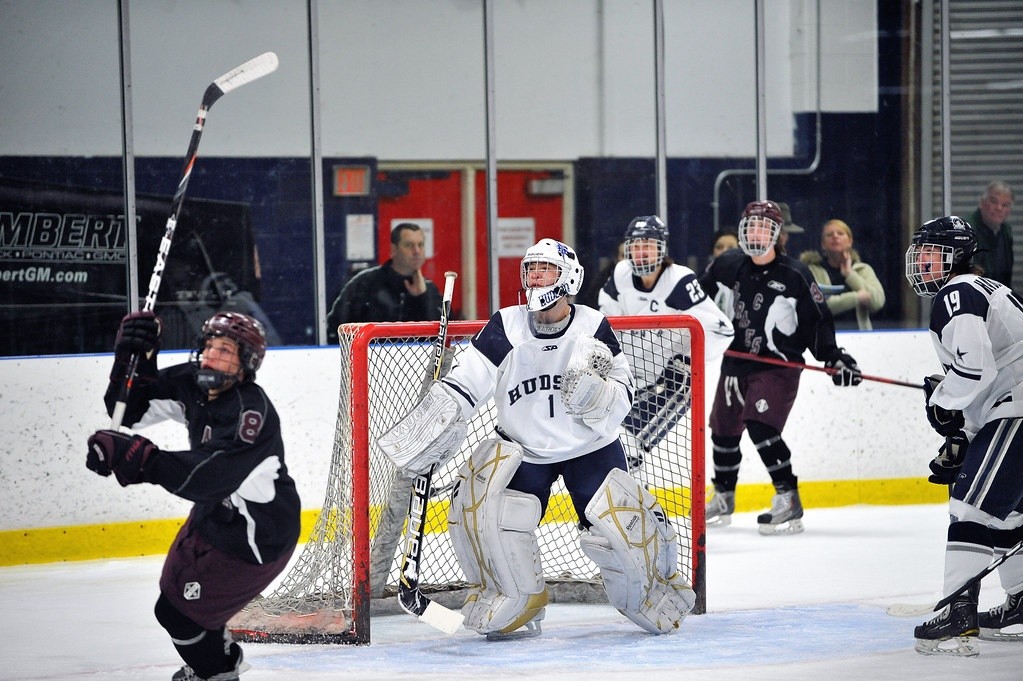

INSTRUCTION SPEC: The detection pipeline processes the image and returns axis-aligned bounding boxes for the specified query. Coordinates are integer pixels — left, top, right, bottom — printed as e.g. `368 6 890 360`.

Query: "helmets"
905 215 978 299
520 238 584 312
624 214 670 276
187 311 266 396
738 200 784 257
202 272 238 309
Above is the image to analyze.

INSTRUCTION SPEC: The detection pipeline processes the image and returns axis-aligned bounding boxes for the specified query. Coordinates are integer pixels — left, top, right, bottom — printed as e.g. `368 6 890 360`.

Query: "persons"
177 272 283 347
577 215 734 532
86 312 301 681
326 223 457 346
965 181 1015 286
379 239 697 640
689 200 862 521
905 215 1023 657
614 202 885 331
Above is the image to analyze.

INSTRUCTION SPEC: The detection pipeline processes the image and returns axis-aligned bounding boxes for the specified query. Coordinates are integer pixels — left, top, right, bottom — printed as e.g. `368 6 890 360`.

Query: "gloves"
826 351 862 387
85 430 157 487
929 435 969 484
922 374 967 435
109 310 165 382
657 354 691 396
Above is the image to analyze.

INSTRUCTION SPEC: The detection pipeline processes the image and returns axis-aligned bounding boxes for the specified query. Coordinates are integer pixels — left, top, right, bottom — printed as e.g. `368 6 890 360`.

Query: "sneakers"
978 591 1023 641
757 476 804 536
914 597 980 656
688 490 735 529
172 628 243 681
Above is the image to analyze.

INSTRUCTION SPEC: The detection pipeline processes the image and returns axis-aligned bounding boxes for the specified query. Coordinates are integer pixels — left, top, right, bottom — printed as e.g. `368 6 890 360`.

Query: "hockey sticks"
933 540 1023 612
724 349 924 389
397 272 466 635
108 51 280 431
